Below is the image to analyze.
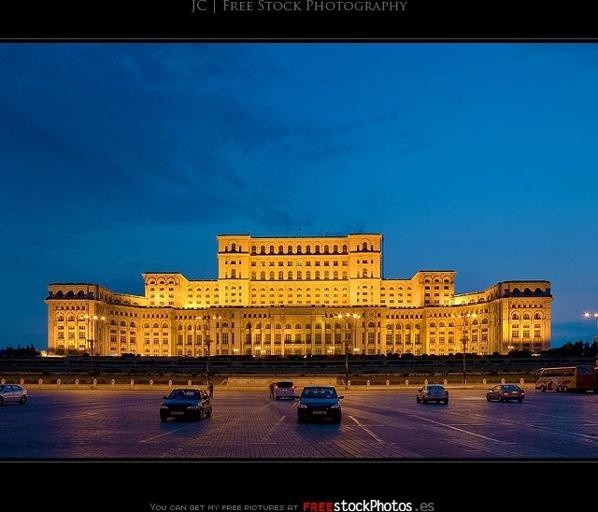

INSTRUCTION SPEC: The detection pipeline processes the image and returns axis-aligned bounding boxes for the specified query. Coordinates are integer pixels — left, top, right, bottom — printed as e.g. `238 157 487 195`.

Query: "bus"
533 365 597 393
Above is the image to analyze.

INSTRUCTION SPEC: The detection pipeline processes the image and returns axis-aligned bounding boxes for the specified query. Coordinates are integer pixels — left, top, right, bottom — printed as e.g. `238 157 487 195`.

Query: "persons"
268 380 276 399
207 382 213 399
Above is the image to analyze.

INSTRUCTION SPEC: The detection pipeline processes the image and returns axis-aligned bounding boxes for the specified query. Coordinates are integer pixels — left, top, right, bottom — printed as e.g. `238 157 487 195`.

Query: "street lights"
193 313 223 386
450 311 479 384
78 312 106 384
331 310 364 386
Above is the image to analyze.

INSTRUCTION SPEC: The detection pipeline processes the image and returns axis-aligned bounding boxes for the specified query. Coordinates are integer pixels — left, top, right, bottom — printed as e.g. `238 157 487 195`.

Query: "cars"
297 385 345 425
268 380 297 401
416 384 449 405
159 388 213 423
0 383 29 405
485 383 526 404
